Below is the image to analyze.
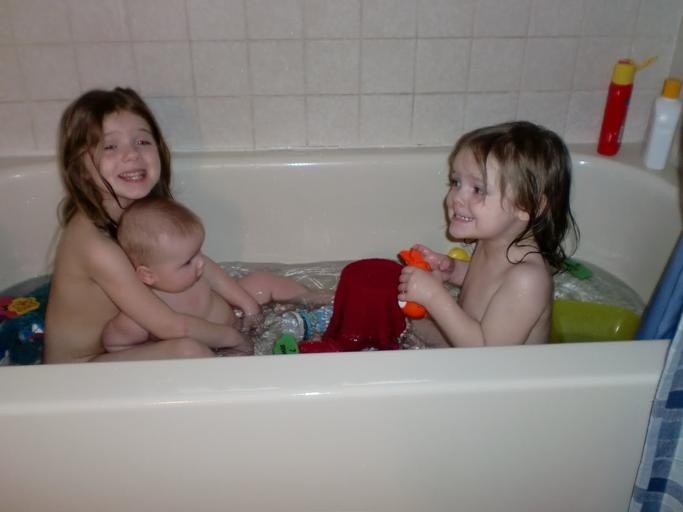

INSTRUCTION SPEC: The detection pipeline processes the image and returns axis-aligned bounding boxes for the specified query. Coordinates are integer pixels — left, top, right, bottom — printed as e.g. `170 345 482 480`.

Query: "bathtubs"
0 140 683 512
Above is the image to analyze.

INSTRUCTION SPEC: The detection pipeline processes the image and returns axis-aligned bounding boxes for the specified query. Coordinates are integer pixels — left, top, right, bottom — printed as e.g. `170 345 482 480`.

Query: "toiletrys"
639 77 683 171
597 54 659 156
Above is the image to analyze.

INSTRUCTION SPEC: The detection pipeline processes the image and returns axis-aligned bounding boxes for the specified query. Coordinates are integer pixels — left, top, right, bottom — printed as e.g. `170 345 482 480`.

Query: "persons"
405 121 580 348
100 195 335 356
41 86 266 365
